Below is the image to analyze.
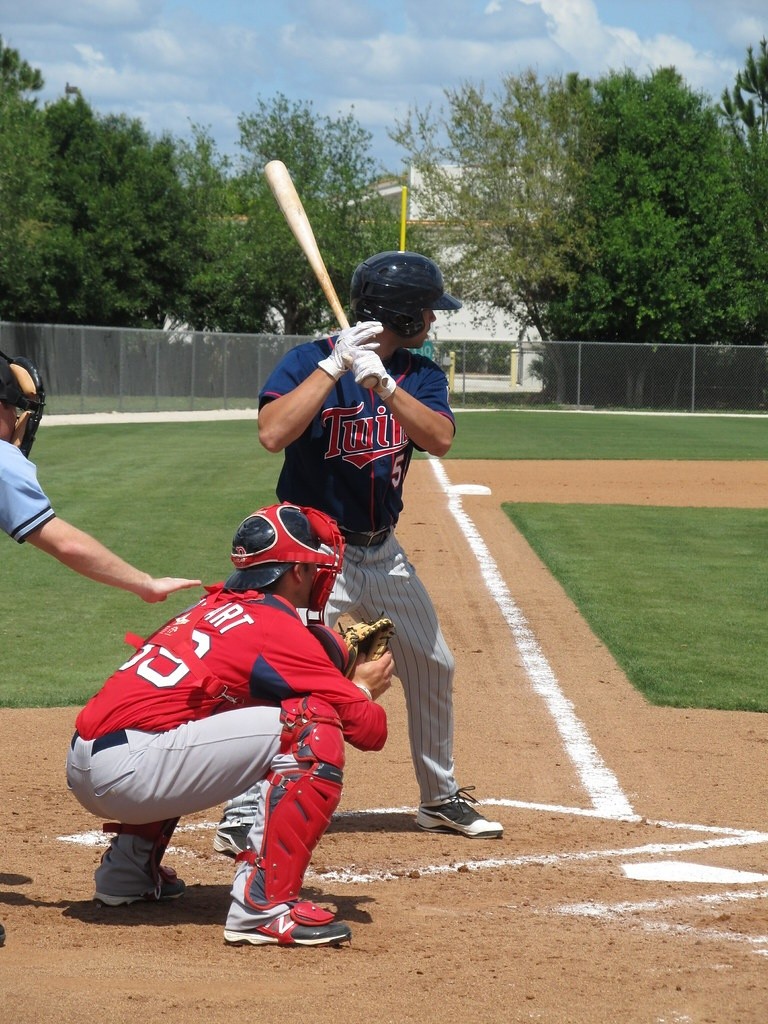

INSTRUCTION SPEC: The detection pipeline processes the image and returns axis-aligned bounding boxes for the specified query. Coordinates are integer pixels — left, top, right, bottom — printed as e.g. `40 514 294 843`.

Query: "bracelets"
356 684 372 700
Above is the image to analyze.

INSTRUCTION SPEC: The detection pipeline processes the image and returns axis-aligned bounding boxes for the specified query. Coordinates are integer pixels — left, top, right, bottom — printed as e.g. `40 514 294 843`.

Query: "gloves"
318 319 383 381
341 349 395 400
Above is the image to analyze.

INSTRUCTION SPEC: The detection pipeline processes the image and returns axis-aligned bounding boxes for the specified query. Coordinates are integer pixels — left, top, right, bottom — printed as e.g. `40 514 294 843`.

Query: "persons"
211 250 506 858
0 349 204 947
64 501 396 948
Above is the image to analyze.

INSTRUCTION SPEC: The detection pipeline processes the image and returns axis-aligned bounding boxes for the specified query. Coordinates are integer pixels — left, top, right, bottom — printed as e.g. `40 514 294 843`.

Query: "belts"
70 726 127 757
336 526 390 547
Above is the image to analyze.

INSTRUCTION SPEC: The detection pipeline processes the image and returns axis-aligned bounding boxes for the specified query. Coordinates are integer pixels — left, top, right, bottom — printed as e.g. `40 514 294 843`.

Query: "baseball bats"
260 157 381 390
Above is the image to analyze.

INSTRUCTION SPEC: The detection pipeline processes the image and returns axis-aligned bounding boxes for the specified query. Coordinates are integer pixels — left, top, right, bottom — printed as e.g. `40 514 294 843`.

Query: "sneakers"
213 814 252 856
94 867 186 905
223 896 352 947
416 785 504 839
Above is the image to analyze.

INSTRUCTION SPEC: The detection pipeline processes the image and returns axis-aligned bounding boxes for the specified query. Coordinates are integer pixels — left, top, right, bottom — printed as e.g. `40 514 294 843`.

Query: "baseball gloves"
335 609 397 683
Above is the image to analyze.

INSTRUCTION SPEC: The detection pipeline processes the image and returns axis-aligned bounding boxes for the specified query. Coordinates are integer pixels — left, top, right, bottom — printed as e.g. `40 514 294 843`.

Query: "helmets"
350 250 461 339
0 348 45 460
222 505 321 589
